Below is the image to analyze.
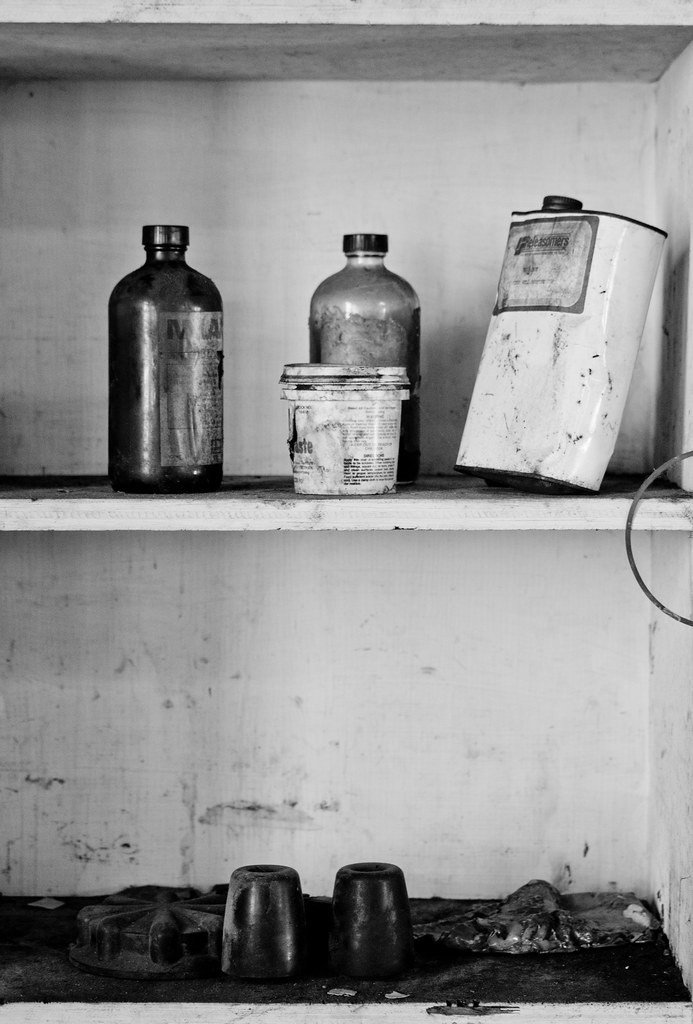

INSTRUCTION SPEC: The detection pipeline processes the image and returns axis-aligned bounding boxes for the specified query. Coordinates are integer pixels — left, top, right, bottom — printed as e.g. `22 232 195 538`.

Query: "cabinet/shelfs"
0 0 693 1024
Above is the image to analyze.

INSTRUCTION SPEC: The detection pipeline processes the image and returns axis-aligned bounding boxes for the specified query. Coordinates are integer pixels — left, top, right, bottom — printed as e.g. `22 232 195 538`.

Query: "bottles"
309 233 425 484
108 225 225 491
453 195 670 491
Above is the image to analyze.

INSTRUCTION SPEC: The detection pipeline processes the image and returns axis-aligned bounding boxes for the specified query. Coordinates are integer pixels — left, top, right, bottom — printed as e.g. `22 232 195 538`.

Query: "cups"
279 362 409 494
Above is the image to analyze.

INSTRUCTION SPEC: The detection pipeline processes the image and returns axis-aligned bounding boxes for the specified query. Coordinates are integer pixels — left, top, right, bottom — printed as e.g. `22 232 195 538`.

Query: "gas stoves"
0 862 693 1004
